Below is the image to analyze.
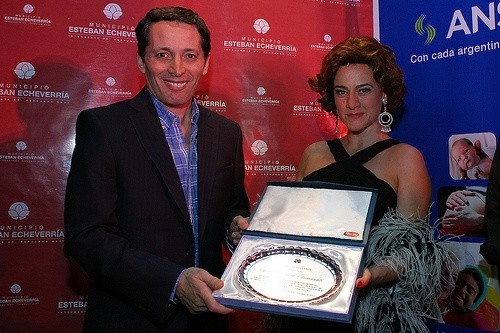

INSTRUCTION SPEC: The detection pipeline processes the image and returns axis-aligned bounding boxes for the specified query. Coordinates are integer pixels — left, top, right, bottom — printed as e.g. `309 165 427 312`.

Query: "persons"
439 137 493 330
257 34 459 333
64 6 251 333
